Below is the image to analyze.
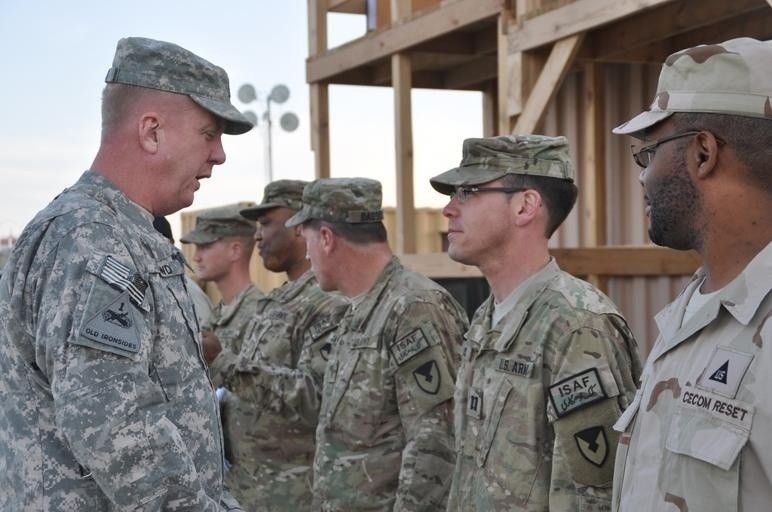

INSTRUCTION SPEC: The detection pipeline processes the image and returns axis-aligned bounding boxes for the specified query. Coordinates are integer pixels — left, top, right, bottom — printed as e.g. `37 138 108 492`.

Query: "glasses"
448 184 531 204
628 128 729 167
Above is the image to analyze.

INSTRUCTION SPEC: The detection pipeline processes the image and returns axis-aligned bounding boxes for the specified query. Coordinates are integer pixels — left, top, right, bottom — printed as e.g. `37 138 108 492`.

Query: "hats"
237 178 311 219
103 36 255 141
427 133 576 197
607 37 772 138
283 175 389 232
179 203 260 246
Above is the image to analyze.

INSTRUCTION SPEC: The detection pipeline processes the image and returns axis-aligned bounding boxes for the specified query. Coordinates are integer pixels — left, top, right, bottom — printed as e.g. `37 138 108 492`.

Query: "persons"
195 175 353 509
429 132 646 509
175 205 271 352
0 33 257 511
599 31 770 512
283 175 471 508
